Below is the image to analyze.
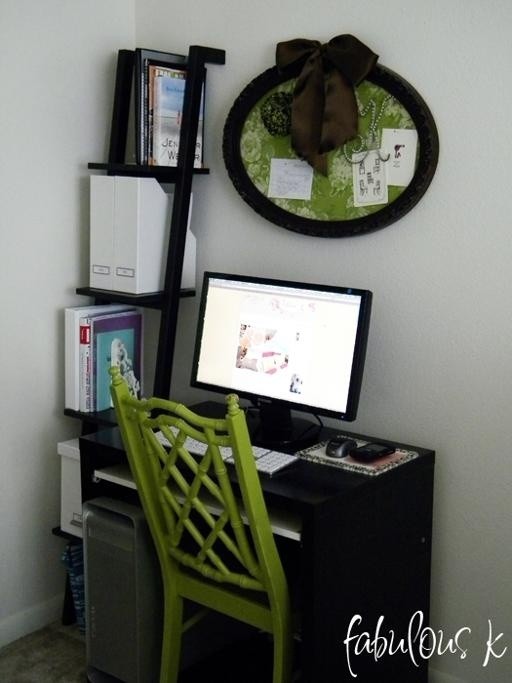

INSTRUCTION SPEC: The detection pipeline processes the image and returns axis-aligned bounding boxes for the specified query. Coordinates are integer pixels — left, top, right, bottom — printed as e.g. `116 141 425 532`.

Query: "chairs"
111 365 290 681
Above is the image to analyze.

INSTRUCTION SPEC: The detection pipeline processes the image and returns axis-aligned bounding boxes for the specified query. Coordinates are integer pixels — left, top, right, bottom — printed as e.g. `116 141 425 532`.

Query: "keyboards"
154 426 298 477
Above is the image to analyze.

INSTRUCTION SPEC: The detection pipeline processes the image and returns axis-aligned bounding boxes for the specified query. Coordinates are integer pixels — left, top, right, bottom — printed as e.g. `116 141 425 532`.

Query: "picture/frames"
222 63 440 239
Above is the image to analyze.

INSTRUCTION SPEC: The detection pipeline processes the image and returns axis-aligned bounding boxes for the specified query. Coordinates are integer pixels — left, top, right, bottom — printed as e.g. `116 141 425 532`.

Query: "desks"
81 402 432 682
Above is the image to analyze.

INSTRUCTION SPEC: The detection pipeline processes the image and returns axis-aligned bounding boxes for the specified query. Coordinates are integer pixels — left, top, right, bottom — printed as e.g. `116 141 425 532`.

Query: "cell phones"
349 441 396 461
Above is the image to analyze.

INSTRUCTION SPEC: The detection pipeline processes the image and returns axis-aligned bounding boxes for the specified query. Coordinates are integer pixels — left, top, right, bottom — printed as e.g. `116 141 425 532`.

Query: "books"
135 48 205 169
64 303 145 413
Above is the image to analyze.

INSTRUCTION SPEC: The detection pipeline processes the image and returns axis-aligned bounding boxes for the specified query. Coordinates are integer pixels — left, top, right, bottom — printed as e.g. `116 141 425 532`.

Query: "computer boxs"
81 493 162 683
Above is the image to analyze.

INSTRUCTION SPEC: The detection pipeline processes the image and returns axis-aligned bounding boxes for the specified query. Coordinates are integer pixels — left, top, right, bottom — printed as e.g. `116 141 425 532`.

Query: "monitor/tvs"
189 270 373 453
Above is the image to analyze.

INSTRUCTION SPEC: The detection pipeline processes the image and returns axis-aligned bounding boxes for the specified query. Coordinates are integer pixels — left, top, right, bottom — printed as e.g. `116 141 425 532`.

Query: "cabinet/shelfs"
53 45 226 644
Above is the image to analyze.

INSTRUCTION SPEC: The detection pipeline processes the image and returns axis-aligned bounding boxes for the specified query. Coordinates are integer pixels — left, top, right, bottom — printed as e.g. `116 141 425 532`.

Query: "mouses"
326 437 357 457
